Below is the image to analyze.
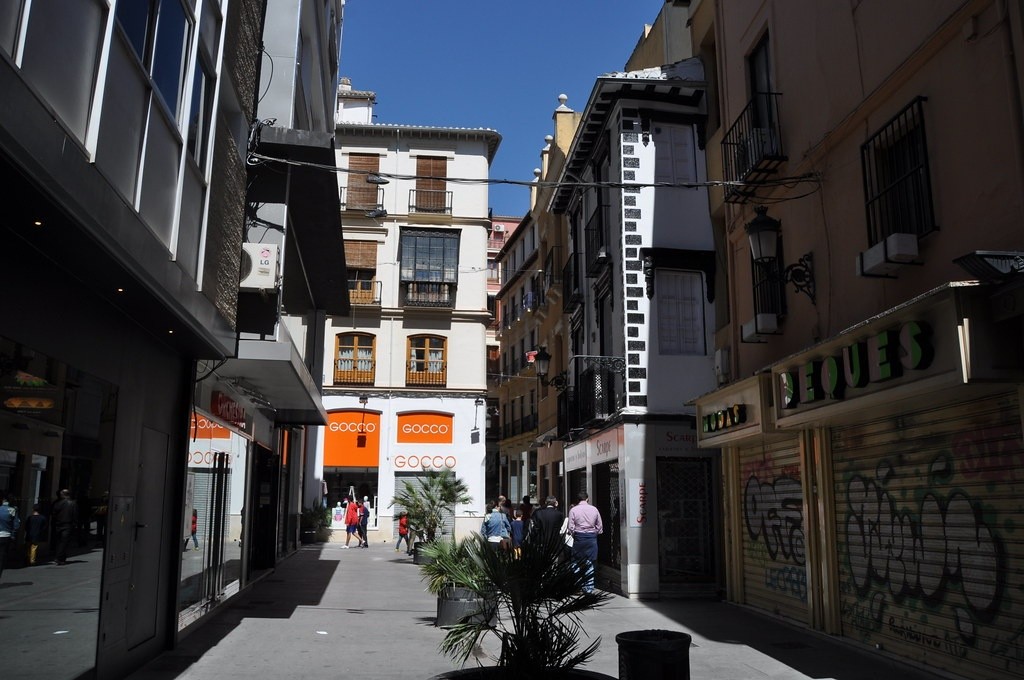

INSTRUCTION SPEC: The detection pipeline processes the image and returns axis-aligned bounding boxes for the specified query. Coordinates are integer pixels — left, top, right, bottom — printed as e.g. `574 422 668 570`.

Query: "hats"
60 489 70 499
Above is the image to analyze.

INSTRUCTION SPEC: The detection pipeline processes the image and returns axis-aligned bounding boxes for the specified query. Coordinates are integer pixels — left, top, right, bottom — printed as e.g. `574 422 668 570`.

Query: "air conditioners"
735 128 774 175
239 242 281 301
494 224 504 233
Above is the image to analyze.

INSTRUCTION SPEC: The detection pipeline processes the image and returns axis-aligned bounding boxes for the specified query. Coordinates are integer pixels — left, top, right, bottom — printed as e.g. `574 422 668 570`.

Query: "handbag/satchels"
500 538 513 554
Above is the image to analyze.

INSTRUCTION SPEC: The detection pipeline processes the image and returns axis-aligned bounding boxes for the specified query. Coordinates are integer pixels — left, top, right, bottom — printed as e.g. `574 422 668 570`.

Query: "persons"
24 485 110 567
341 495 370 549
395 511 425 552
0 498 21 578
184 508 200 552
482 491 603 598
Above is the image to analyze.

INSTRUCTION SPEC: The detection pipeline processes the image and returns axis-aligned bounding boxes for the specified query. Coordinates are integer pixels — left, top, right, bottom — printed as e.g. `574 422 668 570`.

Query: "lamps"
475 397 483 405
744 205 815 306
952 249 1024 280
534 345 567 391
360 393 368 403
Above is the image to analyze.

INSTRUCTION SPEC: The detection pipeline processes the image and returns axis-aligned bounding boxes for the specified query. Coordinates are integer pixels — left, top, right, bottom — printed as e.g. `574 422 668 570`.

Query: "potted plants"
300 497 332 543
416 527 498 628
425 516 620 680
388 465 473 564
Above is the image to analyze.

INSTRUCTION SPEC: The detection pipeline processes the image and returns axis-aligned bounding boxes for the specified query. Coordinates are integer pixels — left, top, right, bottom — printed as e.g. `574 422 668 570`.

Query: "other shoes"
407 550 413 556
54 558 65 565
192 547 199 551
395 548 401 553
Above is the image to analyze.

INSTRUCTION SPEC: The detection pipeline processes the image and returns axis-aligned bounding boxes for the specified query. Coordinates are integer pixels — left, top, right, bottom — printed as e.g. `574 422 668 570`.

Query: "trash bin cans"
615 629 691 680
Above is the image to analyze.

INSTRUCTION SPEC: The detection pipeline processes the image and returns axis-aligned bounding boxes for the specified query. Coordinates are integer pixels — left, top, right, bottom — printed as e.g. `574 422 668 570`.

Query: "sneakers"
360 541 366 549
340 544 350 549
